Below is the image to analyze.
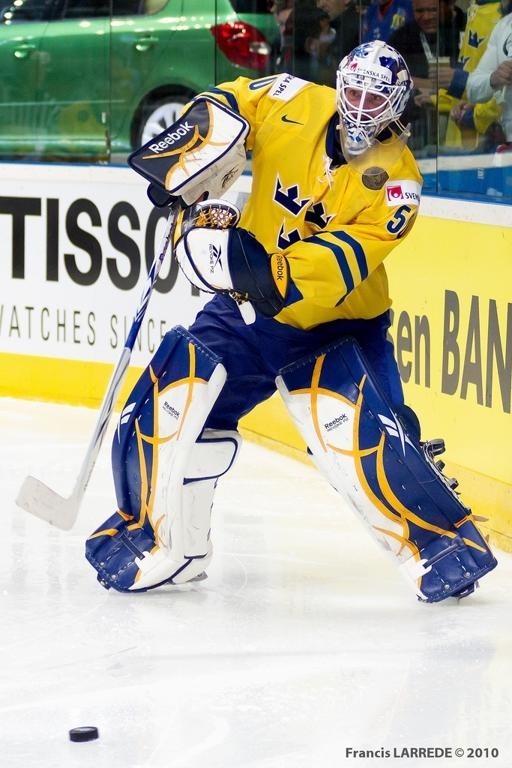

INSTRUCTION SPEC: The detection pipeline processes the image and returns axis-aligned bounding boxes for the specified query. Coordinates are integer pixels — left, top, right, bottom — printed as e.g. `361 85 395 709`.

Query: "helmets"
335 40 414 174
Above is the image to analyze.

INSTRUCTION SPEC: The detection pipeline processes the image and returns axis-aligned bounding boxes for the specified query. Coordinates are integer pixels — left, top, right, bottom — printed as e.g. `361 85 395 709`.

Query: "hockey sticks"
15 197 181 532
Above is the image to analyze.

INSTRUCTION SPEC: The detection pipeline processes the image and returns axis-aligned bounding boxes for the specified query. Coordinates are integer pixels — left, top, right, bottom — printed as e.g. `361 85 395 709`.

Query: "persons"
270 0 512 155
84 38 498 604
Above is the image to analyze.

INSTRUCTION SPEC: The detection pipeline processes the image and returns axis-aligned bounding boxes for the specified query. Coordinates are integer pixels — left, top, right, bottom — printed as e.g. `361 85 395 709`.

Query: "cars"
0 0 282 159
